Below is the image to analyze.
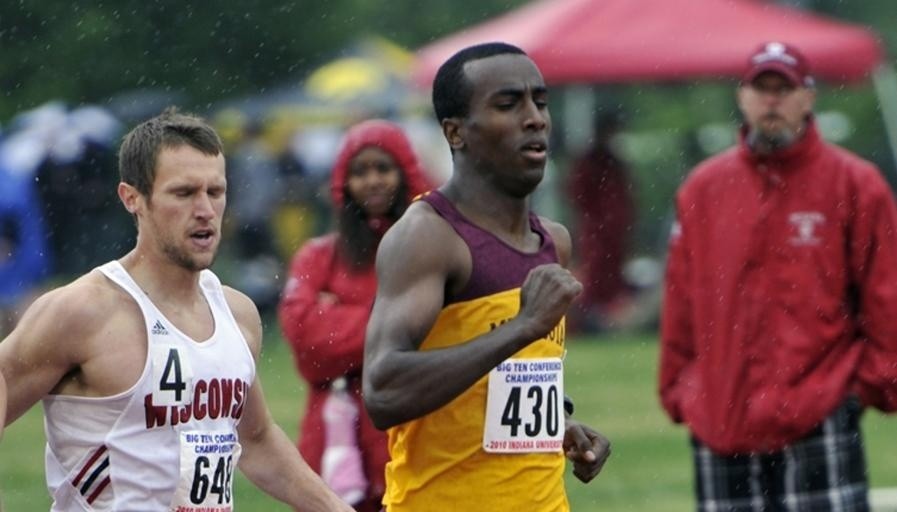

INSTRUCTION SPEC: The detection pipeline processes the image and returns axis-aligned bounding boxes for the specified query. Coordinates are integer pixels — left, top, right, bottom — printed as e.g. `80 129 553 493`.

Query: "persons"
573 134 633 331
657 39 897 511
275 119 436 509
362 43 612 512
0 108 358 512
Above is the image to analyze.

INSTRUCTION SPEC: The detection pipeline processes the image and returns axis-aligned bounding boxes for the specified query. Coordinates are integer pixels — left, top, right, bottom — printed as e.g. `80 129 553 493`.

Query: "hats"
739 41 812 89
